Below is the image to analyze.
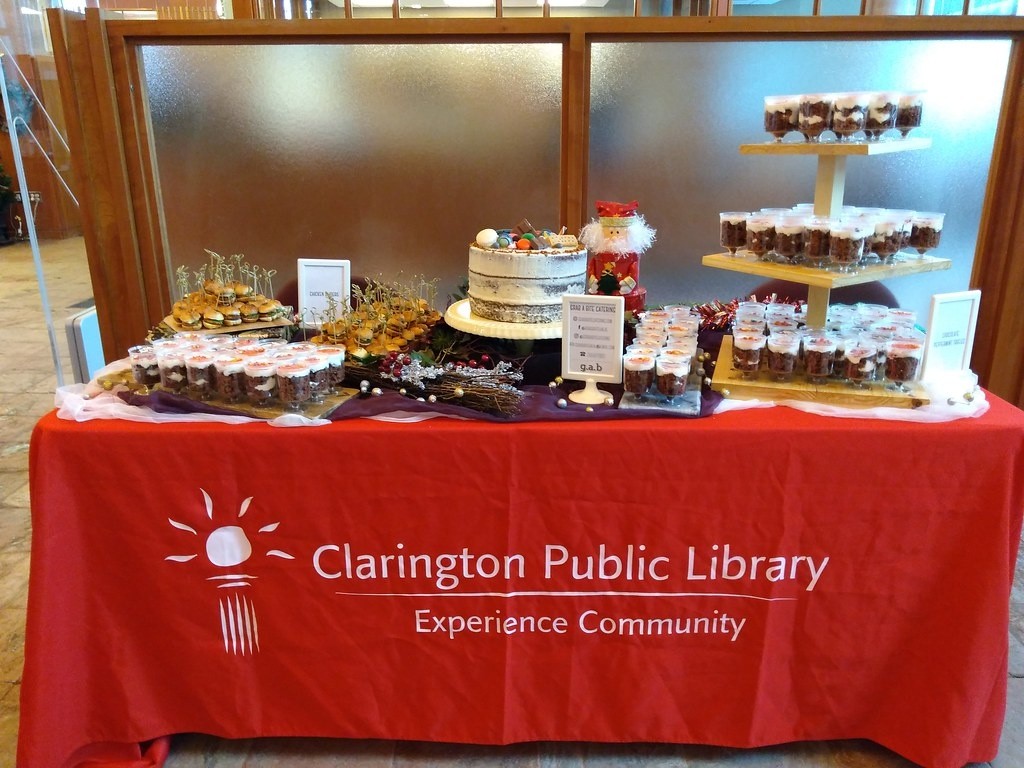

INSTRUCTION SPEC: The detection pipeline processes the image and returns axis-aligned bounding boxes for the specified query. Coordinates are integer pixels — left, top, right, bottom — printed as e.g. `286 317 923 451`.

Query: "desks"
15 385 1024 768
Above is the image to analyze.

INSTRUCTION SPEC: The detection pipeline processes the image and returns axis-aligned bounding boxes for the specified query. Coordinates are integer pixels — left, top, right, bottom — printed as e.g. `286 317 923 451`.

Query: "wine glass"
719 203 946 394
129 331 346 417
624 304 699 407
762 90 925 143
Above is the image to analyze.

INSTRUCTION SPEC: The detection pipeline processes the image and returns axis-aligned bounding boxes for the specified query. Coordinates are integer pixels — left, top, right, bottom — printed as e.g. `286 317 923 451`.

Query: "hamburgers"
172 279 285 330
466 218 589 324
310 296 442 360
